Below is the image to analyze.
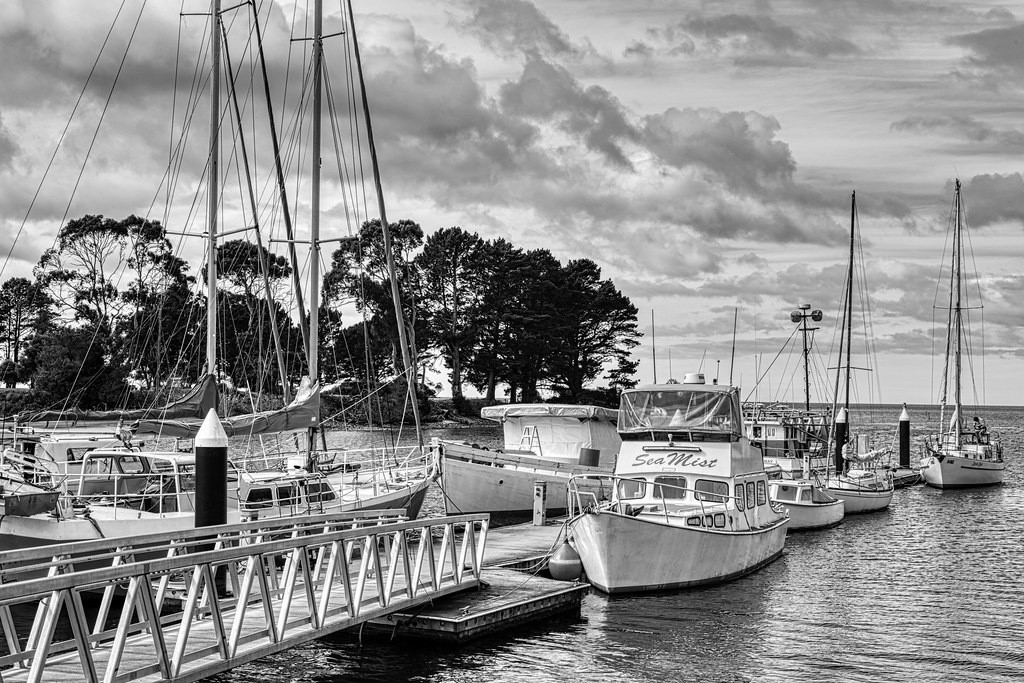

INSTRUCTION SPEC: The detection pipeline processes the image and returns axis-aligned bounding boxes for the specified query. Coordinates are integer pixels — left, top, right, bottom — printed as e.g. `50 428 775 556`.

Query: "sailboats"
1 0 443 615
809 190 895 517
919 176 1005 491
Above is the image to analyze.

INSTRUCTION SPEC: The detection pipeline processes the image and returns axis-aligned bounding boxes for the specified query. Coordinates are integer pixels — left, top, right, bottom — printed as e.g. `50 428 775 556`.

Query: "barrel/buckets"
579 448 600 479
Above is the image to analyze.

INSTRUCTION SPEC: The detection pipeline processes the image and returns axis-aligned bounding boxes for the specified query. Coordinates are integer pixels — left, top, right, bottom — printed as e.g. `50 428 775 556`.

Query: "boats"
565 307 791 595
768 454 844 535
428 402 634 529
741 353 836 458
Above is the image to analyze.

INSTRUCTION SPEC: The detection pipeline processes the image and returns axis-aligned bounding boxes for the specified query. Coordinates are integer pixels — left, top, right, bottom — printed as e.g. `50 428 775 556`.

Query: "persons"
973 417 987 441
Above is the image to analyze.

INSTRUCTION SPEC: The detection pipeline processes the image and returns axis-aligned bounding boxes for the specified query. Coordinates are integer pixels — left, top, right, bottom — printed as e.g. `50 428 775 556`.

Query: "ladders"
515 426 543 471
